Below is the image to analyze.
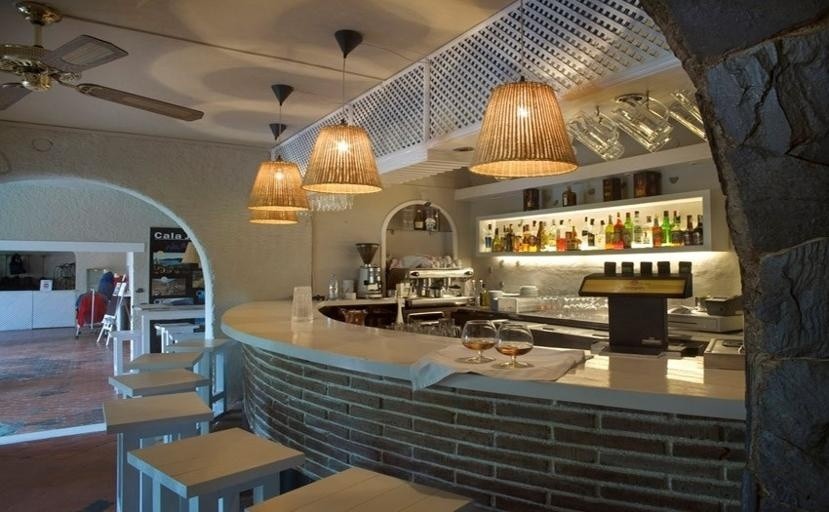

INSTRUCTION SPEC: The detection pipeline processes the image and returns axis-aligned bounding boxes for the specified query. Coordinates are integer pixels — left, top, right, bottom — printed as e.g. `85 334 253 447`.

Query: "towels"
408 342 592 392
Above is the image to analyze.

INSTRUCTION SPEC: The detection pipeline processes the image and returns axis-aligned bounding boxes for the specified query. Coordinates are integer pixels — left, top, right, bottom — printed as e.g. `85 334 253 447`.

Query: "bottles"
479 283 488 307
562 187 579 207
484 210 704 252
328 272 339 301
414 208 426 231
342 280 355 299
469 279 484 306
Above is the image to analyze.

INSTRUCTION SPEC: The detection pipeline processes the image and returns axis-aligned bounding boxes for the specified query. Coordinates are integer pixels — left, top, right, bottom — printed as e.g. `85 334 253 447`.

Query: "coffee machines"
355 243 383 299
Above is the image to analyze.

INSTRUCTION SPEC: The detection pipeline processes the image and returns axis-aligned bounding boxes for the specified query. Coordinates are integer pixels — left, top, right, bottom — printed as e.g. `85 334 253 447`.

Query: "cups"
567 82 708 162
384 317 460 339
604 260 693 276
346 293 357 299
292 286 314 322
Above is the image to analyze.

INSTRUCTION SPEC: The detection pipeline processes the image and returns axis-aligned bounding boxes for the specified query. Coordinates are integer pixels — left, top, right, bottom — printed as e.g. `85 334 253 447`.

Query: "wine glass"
537 294 609 325
493 322 535 371
457 319 496 365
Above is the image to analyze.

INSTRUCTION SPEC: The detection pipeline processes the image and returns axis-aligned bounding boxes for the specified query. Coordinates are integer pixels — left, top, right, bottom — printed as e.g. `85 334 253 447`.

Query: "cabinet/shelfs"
455 141 729 258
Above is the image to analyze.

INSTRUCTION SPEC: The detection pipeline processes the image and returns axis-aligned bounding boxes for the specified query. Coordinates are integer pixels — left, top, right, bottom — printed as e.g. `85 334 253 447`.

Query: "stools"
103 322 214 512
243 466 474 512
126 427 306 512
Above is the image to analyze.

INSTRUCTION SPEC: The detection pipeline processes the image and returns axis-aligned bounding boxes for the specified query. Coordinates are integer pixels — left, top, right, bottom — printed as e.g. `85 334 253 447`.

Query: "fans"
0 0 204 123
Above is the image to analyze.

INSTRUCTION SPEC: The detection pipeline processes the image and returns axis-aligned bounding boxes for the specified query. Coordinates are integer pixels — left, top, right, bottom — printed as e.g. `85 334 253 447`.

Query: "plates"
520 286 538 296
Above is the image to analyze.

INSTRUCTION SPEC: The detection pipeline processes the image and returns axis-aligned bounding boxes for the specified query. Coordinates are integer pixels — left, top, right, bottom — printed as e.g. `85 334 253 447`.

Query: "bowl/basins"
488 290 503 301
429 287 443 298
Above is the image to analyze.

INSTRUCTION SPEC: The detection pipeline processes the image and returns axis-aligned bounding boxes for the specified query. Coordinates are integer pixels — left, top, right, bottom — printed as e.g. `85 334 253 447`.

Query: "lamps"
301 29 383 195
181 242 205 332
469 0 578 179
250 124 301 225
248 84 312 212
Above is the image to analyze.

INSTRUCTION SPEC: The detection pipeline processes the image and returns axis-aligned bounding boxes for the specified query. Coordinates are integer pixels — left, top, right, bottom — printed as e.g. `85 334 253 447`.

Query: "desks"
133 305 205 355
